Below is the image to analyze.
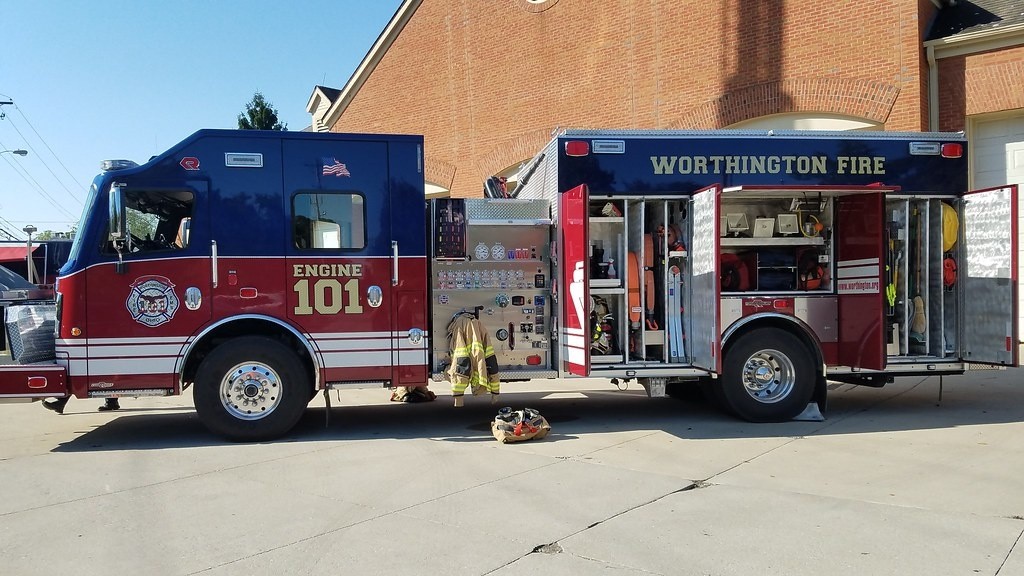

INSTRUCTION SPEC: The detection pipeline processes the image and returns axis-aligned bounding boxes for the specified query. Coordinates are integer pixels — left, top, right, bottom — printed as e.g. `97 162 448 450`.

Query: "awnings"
0 242 41 262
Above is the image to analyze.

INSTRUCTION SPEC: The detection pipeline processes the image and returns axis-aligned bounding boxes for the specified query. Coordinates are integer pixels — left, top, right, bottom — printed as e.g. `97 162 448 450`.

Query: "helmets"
799 262 824 290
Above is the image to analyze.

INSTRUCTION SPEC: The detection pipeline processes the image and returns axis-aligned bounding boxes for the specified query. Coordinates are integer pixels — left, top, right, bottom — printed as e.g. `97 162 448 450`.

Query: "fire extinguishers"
602 313 615 342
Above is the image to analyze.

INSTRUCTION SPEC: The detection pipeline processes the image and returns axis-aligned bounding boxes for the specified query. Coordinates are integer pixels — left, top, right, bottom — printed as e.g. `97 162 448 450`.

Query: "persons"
42 394 120 414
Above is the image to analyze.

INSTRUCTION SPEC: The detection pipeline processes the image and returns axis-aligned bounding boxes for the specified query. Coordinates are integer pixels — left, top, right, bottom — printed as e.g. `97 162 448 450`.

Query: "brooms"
913 210 925 333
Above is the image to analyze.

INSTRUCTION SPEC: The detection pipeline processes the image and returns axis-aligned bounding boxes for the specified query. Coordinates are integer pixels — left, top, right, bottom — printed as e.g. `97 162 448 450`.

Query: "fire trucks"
0 128 1019 445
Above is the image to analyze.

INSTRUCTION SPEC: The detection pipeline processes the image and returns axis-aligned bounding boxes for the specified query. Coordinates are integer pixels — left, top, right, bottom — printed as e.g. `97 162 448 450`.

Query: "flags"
322 157 346 176
336 169 350 178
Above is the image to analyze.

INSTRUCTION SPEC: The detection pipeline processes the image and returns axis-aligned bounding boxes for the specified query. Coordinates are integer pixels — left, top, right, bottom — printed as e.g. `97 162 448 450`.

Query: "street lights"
21 224 37 284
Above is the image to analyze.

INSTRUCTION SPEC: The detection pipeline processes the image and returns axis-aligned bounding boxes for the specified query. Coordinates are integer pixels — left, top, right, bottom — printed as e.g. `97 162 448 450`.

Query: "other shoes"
42 400 65 414
98 402 119 411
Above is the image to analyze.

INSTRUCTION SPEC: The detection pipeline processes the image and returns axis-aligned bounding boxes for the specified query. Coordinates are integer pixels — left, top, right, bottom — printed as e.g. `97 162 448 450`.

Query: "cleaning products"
608 257 616 278
